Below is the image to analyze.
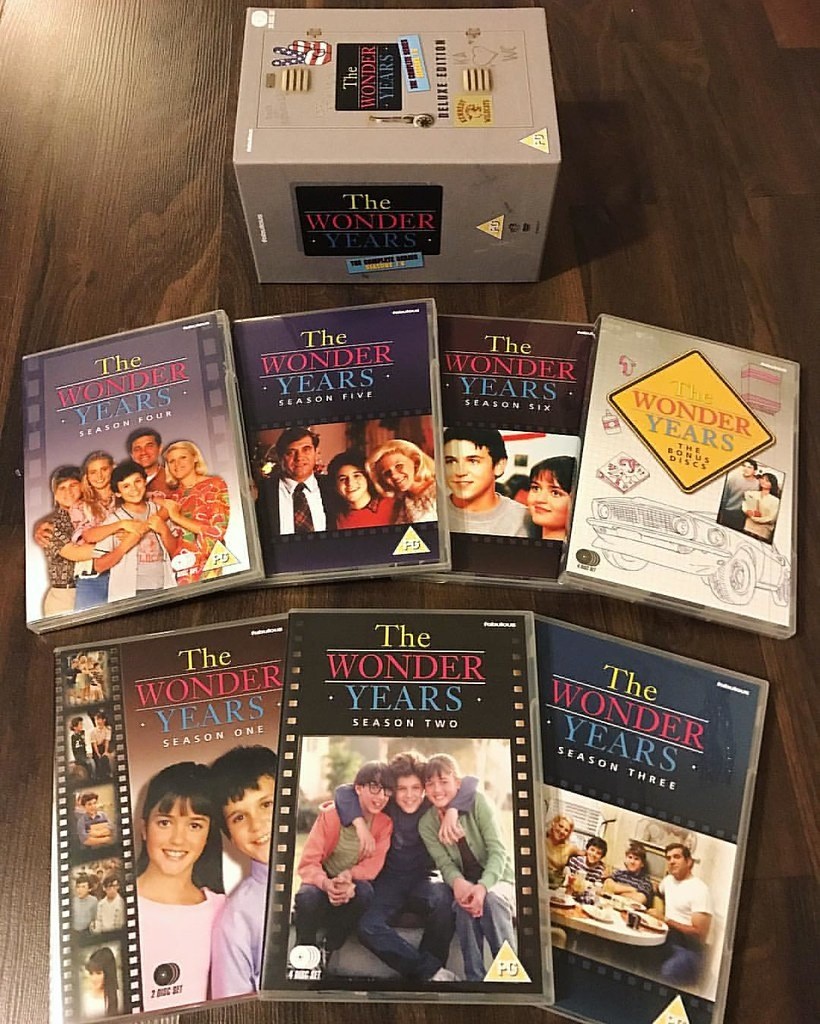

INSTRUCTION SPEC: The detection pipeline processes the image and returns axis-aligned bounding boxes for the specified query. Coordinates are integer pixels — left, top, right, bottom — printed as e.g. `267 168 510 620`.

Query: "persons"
33 428 230 617
530 453 579 542
256 427 439 537
443 418 539 540
544 814 712 985
213 746 275 997
291 749 518 981
720 458 780 540
131 746 230 1011
59 648 127 1018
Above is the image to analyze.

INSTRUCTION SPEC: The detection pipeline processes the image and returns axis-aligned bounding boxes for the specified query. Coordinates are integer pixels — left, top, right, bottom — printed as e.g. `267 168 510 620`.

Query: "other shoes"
431 970 458 982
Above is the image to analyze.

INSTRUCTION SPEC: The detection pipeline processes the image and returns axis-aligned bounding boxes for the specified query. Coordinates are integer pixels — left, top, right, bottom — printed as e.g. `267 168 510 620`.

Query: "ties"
292 482 315 533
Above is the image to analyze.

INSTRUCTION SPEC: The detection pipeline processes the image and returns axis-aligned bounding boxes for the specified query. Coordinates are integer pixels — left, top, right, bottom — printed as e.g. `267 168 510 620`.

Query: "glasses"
364 780 393 798
